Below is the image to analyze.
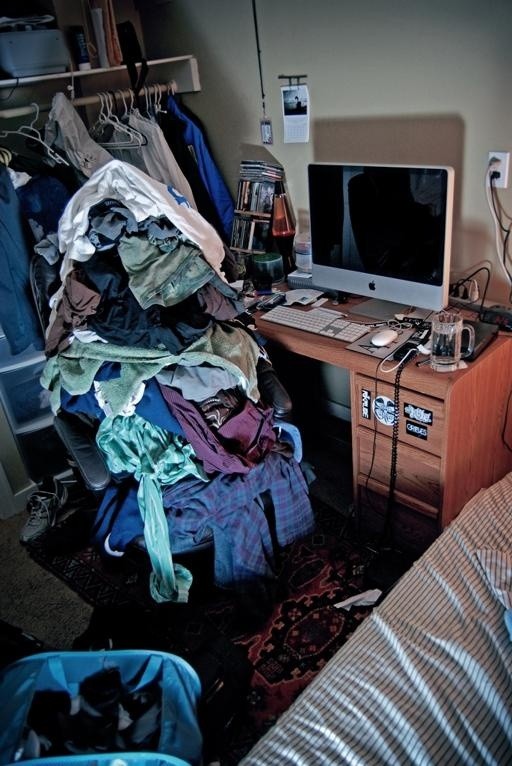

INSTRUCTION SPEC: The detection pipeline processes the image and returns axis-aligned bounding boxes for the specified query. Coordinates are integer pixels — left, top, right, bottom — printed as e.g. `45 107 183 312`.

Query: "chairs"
0 650 201 763
30 240 303 566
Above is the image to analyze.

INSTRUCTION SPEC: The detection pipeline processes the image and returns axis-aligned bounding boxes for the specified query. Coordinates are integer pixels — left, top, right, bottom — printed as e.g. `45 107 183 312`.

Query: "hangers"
0 103 69 167
88 82 175 149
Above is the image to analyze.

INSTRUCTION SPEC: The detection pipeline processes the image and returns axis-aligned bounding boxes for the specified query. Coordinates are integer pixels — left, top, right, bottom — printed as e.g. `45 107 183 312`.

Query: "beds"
236 468 512 766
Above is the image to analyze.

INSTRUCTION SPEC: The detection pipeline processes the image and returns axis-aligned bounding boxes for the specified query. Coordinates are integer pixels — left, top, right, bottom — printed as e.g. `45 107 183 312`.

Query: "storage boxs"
253 254 285 284
295 251 312 268
0 29 69 76
288 271 312 288
0 338 66 433
295 241 311 254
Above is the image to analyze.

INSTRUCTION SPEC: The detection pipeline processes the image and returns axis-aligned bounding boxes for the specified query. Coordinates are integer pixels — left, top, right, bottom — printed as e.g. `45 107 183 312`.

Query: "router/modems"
430 318 498 361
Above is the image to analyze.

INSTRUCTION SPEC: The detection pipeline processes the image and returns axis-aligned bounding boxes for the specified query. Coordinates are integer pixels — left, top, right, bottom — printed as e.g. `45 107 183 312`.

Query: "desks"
251 281 512 532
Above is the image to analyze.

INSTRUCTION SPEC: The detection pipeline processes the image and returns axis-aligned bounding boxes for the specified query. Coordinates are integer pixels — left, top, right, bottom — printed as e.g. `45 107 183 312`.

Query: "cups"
431 313 475 372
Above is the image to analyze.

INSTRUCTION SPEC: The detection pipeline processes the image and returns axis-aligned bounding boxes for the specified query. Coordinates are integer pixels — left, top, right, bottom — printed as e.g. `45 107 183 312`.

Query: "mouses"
372 329 398 347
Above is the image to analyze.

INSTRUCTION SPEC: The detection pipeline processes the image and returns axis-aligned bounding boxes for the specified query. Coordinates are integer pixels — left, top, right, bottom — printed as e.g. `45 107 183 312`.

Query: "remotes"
255 291 285 310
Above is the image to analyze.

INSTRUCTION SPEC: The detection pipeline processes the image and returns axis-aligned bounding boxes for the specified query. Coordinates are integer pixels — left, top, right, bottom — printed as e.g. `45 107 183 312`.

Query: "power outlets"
450 295 502 313
488 152 510 187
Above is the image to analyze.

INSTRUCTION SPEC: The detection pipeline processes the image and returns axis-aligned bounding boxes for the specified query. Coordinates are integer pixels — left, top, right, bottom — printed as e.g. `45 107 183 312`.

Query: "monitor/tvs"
307 162 456 321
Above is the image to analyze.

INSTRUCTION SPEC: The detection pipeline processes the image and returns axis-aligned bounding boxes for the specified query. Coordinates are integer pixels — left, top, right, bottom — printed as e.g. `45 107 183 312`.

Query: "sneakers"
19 477 67 545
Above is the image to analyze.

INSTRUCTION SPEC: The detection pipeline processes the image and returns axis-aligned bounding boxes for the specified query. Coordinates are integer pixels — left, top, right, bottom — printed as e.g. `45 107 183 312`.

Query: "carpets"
23 511 437 766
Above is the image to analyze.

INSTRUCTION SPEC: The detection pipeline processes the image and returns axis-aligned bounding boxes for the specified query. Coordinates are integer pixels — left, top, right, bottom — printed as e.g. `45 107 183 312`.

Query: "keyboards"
259 305 370 343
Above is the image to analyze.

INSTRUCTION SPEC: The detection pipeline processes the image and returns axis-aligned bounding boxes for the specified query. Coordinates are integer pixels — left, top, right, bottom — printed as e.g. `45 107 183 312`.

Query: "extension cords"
448 290 509 313
